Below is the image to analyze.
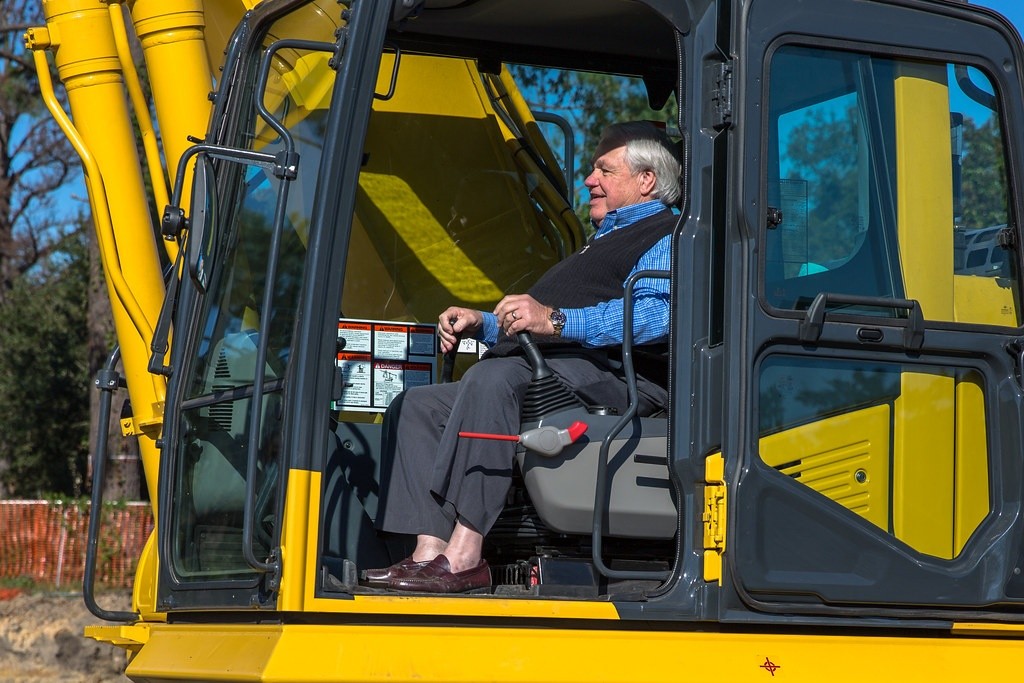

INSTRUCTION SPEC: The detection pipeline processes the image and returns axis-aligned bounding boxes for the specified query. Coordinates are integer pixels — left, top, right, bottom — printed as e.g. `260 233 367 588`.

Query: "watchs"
548 305 567 336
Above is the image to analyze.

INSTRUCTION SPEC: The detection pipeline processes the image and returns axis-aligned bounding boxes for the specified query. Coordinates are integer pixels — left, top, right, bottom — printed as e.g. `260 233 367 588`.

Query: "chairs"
488 378 675 542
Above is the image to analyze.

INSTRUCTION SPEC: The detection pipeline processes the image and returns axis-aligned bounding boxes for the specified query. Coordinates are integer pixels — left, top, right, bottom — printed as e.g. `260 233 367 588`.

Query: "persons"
359 120 685 594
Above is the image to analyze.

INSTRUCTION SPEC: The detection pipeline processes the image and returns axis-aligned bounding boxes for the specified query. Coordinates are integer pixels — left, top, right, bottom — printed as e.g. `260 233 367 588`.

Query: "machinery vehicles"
17 1 1024 682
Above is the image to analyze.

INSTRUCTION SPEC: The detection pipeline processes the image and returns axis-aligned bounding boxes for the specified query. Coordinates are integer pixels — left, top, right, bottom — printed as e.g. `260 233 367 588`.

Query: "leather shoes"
360 554 492 595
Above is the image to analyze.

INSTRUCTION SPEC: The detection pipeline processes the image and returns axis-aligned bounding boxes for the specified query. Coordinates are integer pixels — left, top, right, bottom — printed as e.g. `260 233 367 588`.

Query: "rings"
511 310 518 320
